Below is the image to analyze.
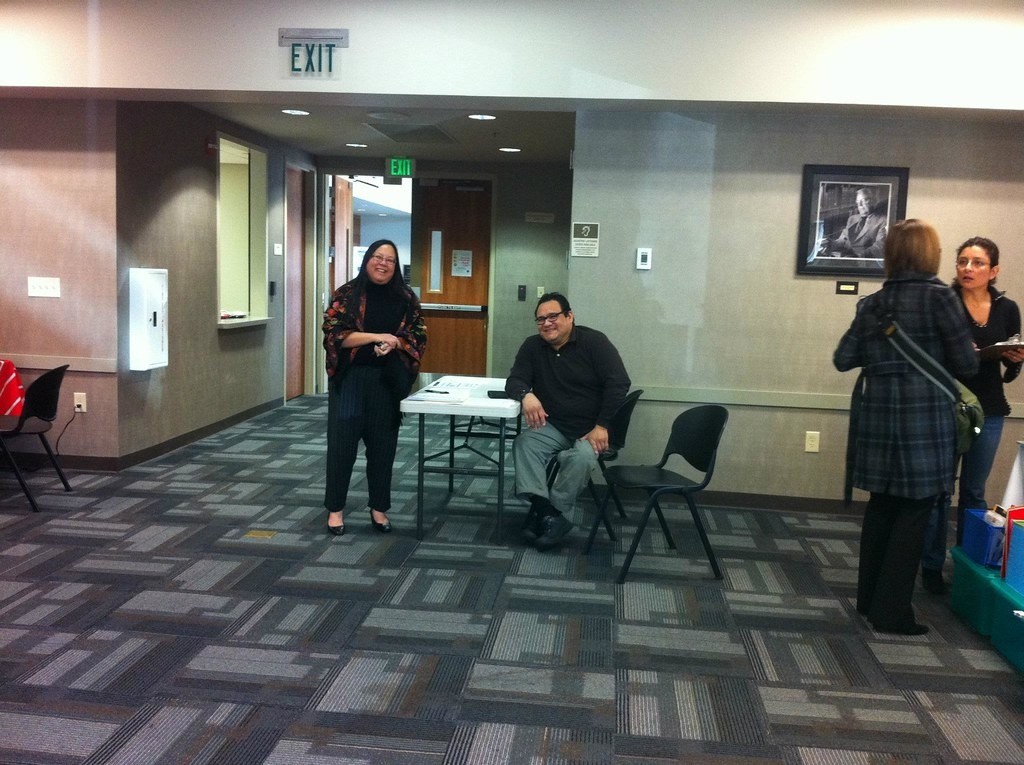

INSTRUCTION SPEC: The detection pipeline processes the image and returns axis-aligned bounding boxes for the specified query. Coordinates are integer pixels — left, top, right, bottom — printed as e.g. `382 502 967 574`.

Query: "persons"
505 292 631 552
322 239 428 536
833 219 978 637
921 236 1024 600
820 188 886 257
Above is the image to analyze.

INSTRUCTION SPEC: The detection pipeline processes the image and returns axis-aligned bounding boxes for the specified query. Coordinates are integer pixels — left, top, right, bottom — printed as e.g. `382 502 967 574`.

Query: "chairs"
0 364 72 513
524 389 643 543
585 404 729 584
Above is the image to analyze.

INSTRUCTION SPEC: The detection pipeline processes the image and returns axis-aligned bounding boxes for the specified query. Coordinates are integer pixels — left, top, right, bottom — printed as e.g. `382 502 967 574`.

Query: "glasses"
956 256 994 268
371 254 397 264
535 310 567 325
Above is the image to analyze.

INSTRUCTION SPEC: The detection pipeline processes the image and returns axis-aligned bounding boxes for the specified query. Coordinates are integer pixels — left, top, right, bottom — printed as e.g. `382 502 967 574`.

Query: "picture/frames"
796 164 909 278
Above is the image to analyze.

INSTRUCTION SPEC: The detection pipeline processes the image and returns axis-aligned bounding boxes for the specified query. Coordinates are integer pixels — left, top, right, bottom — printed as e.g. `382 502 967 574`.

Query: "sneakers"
523 504 543 539
536 513 573 548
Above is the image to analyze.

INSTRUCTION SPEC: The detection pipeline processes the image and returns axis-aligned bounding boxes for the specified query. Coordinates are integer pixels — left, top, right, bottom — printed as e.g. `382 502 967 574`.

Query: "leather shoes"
370 509 392 532
328 512 344 535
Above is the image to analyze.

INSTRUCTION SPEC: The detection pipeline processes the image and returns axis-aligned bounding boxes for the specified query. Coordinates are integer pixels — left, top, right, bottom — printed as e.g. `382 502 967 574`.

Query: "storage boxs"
949 505 1024 672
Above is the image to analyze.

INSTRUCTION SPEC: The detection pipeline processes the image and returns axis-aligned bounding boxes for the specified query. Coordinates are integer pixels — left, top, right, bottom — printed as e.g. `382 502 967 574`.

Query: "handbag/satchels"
953 384 983 453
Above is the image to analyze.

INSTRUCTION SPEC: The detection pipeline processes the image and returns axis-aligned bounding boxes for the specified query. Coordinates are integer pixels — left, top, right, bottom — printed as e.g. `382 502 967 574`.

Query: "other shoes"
857 602 875 610
874 615 929 635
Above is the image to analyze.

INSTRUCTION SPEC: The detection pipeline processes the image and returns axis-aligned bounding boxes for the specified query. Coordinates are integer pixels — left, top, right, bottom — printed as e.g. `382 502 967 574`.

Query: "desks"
0 360 26 417
400 374 523 542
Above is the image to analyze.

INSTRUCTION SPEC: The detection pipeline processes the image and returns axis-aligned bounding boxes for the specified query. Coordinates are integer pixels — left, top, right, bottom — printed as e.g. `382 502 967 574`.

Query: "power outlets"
804 431 819 452
73 392 87 413
